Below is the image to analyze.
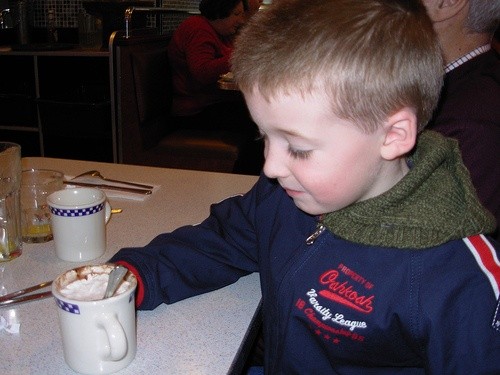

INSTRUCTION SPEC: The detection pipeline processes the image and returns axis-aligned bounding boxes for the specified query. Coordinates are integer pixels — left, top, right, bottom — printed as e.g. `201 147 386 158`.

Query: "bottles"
77 9 103 50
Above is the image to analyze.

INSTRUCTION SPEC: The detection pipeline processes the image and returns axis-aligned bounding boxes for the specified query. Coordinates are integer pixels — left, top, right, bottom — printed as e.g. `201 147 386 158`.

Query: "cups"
47 187 107 262
51 264 137 375
18 169 64 243
0 176 23 261
0 142 22 242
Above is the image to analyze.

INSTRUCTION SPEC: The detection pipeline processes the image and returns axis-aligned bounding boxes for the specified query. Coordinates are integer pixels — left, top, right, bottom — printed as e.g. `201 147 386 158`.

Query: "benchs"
117 39 242 173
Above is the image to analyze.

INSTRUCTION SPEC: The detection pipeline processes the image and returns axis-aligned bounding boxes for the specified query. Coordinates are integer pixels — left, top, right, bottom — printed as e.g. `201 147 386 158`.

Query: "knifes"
63 181 152 194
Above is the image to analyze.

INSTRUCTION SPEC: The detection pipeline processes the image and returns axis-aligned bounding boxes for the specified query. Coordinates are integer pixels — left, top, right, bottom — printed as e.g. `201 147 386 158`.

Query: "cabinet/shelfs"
0 55 110 161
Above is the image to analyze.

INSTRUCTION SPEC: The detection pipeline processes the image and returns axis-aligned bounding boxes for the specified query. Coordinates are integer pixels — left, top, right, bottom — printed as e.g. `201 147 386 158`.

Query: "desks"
0 157 262 375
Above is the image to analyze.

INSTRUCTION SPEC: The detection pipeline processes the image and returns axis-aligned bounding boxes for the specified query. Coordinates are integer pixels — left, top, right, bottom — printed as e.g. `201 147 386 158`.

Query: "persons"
106 0 500 375
421 0 500 243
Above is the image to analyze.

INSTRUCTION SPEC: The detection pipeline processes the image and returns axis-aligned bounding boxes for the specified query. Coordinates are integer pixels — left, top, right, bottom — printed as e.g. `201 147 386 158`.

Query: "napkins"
61 171 162 203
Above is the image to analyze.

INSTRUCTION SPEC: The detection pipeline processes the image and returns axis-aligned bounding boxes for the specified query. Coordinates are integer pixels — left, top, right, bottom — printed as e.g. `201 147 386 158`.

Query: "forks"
71 170 153 189
